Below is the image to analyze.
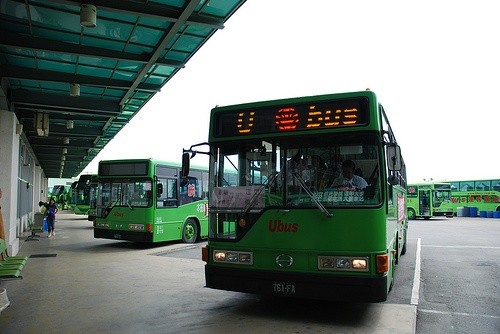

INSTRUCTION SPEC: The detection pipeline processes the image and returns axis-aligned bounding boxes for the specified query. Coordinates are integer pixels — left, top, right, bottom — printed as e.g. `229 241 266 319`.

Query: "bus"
181 88 408 302
94 160 209 244
47 175 98 219
405 178 500 219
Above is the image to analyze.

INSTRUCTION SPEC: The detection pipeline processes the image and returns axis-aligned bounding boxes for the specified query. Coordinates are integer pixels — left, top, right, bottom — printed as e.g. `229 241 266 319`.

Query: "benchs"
25 219 43 240
0 238 28 281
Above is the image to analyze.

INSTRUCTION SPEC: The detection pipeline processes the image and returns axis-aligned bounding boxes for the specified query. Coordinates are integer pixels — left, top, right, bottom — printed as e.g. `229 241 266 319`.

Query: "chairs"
353 167 363 177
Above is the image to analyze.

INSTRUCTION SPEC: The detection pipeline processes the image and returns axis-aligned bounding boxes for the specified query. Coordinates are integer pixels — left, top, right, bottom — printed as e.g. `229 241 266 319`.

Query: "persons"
93 198 98 209
39 199 58 238
330 160 368 191
421 193 428 215
288 154 329 183
61 201 66 210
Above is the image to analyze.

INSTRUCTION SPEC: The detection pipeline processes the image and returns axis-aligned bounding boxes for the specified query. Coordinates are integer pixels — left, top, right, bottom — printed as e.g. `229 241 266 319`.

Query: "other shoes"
48 234 51 237
52 232 55 236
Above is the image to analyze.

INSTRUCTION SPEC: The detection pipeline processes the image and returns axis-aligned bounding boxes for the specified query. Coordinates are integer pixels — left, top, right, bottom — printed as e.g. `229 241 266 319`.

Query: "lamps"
70 83 81 96
66 120 73 129
59 148 67 177
64 137 70 144
80 3 96 28
